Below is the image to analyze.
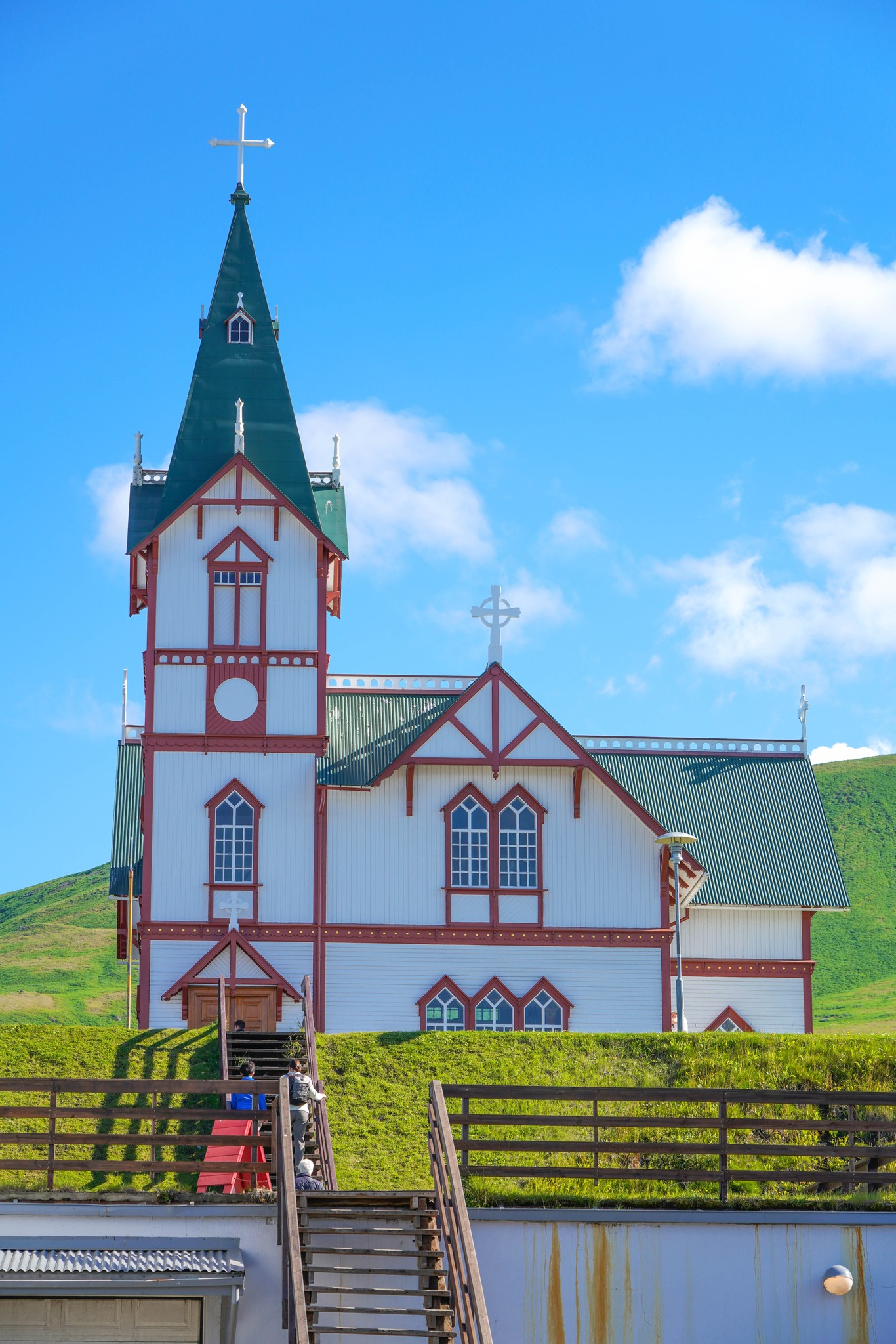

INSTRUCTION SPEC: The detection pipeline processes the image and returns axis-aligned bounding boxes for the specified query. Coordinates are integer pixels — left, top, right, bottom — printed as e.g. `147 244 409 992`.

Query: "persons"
231 1060 268 1131
234 1020 246 1032
275 1059 326 1177
294 1158 325 1191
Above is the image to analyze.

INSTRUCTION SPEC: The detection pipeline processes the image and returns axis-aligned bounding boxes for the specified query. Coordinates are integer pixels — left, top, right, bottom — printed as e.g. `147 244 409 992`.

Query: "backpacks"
285 1073 310 1105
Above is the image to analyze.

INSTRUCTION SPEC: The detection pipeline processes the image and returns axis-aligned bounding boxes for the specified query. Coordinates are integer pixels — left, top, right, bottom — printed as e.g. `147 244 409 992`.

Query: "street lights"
655 831 698 1034
